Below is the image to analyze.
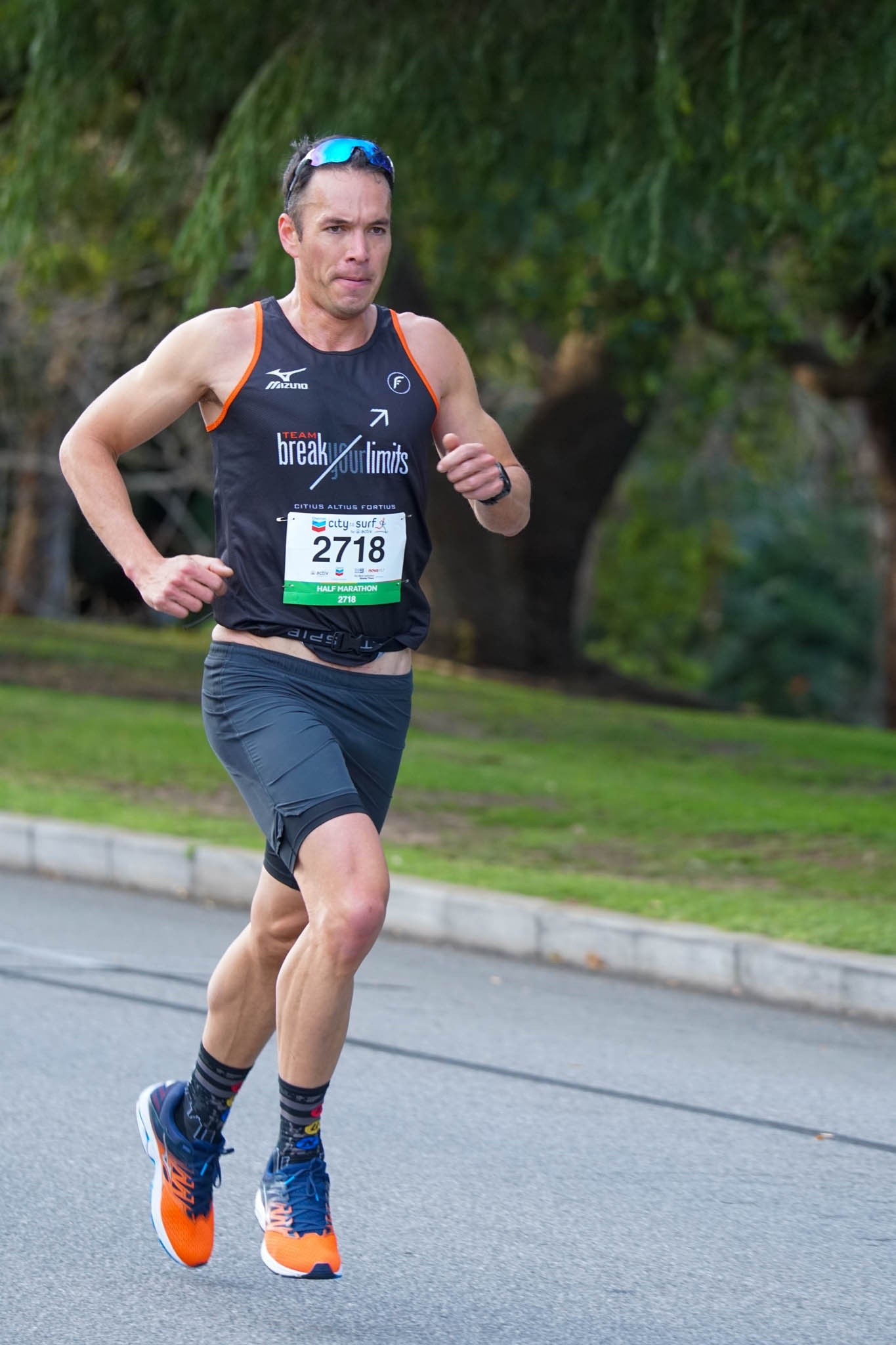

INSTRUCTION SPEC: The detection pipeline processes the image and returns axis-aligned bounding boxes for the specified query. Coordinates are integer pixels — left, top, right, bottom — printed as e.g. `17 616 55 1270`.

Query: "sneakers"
253 1150 343 1279
135 1080 235 1269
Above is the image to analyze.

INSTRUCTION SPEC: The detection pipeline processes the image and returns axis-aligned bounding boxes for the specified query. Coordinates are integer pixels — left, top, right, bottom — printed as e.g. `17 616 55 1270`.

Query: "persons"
58 135 530 1277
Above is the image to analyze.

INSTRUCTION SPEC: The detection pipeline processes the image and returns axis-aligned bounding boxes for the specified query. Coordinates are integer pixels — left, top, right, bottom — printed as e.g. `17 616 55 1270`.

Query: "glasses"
283 138 395 216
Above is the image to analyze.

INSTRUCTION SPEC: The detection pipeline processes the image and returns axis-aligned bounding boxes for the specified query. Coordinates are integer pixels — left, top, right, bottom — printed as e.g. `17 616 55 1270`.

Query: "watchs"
478 461 511 505
